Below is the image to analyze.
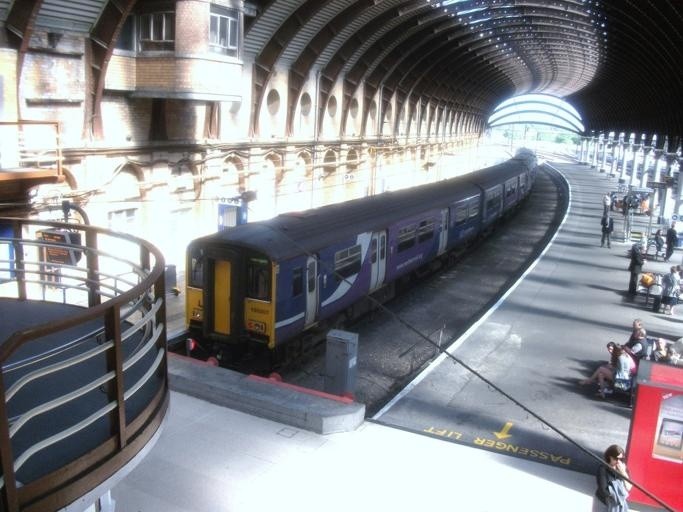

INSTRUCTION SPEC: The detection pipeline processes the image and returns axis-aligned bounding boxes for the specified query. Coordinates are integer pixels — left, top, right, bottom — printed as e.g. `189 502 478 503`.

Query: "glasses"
613 456 625 463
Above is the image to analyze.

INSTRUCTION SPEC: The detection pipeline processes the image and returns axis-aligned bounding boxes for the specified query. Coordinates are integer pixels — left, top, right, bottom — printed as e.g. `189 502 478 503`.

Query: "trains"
184 147 537 368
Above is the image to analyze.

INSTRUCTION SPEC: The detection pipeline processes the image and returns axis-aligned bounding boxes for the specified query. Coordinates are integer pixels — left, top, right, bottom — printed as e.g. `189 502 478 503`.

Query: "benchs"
636 272 663 312
610 338 654 406
642 239 667 262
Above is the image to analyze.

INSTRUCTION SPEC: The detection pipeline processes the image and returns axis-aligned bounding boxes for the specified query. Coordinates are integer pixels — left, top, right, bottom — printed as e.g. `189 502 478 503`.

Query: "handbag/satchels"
595 482 621 506
640 272 654 287
613 379 630 392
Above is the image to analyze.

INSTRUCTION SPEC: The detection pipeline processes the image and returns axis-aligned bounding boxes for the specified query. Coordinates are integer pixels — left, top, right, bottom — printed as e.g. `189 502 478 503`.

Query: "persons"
592 443 633 512
578 191 683 399
